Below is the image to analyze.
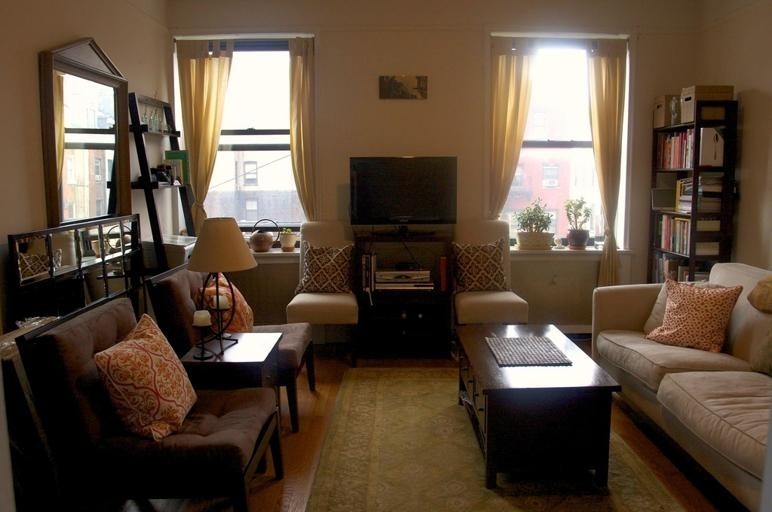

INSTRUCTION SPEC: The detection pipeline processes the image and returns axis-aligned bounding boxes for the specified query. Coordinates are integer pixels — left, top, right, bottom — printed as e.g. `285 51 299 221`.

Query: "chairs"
146 267 317 434
15 296 286 512
286 222 357 369
452 221 529 325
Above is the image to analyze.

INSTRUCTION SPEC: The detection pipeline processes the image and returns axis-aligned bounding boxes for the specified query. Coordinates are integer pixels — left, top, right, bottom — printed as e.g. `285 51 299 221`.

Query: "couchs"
593 263 771 509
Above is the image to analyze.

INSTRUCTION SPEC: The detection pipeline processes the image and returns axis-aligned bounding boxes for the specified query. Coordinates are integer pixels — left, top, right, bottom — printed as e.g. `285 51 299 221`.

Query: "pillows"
294 240 354 295
452 236 510 292
643 279 742 352
93 313 199 443
200 272 254 334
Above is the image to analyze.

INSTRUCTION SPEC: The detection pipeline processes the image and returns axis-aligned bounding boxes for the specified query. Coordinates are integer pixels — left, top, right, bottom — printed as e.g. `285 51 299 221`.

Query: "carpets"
303 367 686 511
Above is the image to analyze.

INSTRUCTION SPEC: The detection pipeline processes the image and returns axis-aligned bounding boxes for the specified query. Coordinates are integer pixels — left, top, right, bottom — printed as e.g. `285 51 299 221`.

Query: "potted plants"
514 197 555 251
566 198 591 249
279 229 297 252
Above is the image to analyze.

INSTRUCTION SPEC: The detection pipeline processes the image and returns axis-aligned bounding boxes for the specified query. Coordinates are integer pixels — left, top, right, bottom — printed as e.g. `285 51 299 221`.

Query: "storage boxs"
654 96 681 128
681 86 733 123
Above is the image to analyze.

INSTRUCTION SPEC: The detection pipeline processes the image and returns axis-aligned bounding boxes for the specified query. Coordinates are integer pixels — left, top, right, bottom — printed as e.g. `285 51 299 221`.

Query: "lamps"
186 218 257 359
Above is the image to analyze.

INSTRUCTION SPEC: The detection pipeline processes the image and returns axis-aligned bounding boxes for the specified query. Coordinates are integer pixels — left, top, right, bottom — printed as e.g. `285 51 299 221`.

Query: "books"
650 126 726 283
162 149 189 185
436 255 448 292
361 252 377 292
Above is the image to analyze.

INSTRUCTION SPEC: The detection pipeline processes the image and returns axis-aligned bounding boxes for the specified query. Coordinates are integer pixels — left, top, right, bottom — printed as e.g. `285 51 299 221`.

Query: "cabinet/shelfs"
128 90 197 272
648 99 738 283
357 292 452 357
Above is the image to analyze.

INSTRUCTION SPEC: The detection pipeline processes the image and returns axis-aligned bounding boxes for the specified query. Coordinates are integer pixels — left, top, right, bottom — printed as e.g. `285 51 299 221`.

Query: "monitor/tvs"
349 156 458 243
